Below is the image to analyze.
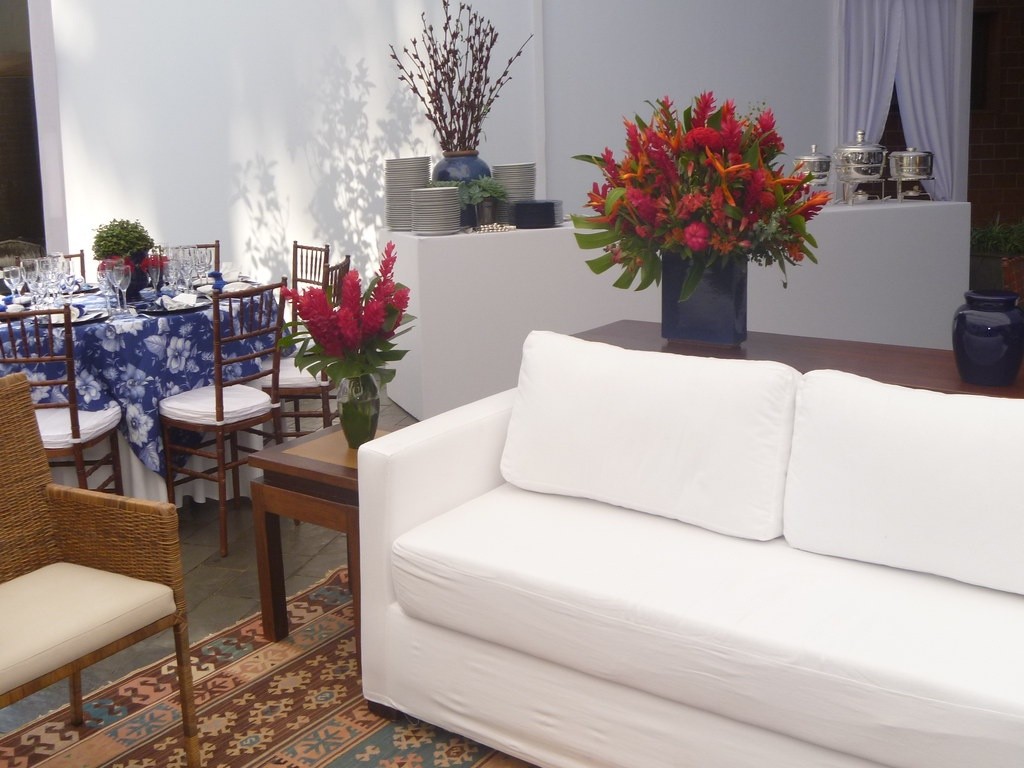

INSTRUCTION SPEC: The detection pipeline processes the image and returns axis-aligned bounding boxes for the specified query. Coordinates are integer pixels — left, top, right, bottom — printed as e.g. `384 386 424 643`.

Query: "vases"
658 250 748 349
148 268 164 287
953 289 1024 386
337 373 380 449
432 150 491 226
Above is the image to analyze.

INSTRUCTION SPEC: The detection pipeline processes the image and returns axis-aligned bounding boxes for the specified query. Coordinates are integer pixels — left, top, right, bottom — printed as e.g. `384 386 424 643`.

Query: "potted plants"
969 210 1024 313
92 218 154 297
433 174 508 223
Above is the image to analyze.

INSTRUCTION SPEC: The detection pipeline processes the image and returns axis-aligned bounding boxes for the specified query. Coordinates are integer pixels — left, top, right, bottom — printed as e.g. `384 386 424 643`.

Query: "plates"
62 284 99 294
30 307 110 327
491 161 537 225
143 297 212 313
190 275 251 286
385 154 462 236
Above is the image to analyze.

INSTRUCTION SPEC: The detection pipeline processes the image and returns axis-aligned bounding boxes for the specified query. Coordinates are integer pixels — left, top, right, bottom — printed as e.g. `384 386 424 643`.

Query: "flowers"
97 256 134 272
142 256 169 272
564 89 833 300
389 0 533 153
277 240 417 388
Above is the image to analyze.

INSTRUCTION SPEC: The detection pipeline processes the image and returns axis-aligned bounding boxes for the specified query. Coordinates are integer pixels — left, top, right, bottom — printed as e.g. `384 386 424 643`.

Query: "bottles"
951 288 1024 387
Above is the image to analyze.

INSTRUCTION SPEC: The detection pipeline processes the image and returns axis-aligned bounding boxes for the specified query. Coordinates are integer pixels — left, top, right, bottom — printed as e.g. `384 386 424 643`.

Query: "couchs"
357 330 1024 768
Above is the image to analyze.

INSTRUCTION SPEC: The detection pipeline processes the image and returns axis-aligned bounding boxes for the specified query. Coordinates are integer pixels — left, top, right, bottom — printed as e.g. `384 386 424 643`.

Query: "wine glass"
96 259 137 321
2 249 77 311
146 243 212 311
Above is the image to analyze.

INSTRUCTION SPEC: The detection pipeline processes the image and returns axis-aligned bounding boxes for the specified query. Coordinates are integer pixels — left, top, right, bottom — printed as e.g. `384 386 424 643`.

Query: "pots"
792 144 831 183
887 147 934 179
832 131 889 180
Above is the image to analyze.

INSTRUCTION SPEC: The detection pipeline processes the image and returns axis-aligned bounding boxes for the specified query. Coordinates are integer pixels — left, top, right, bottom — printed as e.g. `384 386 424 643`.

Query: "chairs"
0 240 351 768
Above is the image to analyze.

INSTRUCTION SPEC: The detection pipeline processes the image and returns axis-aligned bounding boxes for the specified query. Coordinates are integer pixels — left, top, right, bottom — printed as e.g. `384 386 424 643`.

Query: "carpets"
0 563 541 768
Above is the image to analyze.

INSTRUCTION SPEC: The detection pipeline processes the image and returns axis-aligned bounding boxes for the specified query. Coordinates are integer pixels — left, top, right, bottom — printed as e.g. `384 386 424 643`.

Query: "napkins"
220 262 240 282
155 293 197 310
0 304 25 312
0 294 31 304
52 303 87 323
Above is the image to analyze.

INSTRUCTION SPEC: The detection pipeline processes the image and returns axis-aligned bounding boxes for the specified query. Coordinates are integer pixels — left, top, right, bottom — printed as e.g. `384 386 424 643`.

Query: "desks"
0 282 297 509
248 424 393 663
569 319 1024 398
381 200 970 422
